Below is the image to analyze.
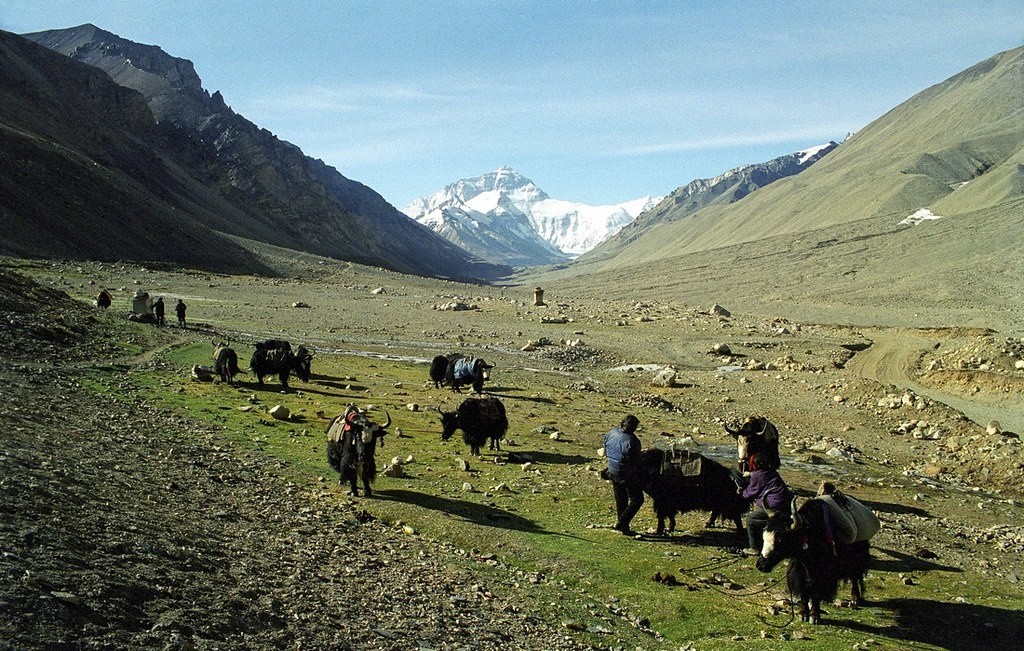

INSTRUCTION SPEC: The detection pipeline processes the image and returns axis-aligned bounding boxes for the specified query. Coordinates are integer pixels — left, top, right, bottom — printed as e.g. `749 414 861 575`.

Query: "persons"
603 415 644 535
743 453 791 556
175 299 187 328
152 298 165 324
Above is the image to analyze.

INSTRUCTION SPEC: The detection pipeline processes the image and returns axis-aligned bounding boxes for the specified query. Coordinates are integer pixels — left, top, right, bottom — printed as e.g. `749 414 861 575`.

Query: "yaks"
429 351 509 456
97 289 112 309
211 337 316 387
599 417 880 624
324 405 392 497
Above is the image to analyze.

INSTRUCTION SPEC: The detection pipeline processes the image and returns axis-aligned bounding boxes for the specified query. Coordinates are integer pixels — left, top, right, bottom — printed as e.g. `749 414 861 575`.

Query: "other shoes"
613 521 630 533
742 547 760 556
